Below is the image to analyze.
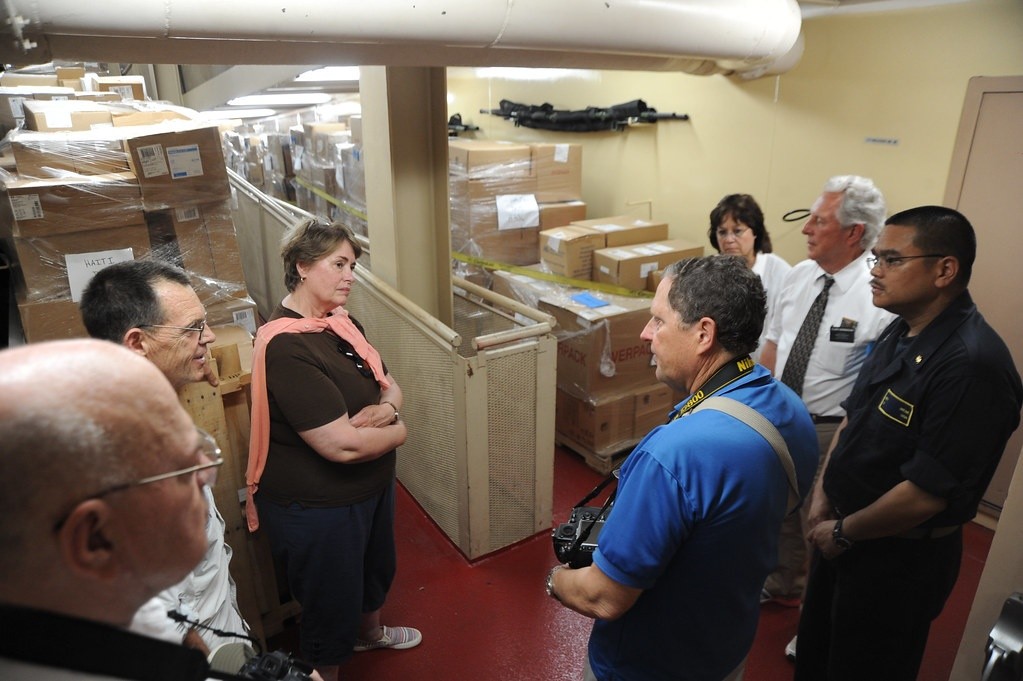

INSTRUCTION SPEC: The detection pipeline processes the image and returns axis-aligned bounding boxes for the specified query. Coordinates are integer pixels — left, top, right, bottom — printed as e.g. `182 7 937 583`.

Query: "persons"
756 175 901 612
546 252 819 681
794 207 1023 681
706 191 791 363
78 259 258 663
0 336 315 681
242 214 424 681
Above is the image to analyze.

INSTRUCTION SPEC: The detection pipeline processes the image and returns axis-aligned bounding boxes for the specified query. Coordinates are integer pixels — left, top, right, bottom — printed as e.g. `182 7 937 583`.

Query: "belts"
810 413 843 424
827 512 959 540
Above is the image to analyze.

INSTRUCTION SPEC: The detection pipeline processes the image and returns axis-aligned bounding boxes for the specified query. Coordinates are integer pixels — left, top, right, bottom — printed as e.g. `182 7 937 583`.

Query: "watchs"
546 564 570 599
831 519 854 553
381 401 400 424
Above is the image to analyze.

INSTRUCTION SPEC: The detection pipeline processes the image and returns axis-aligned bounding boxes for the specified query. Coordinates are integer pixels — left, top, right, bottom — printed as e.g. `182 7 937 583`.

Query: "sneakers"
351 625 422 652
759 586 801 607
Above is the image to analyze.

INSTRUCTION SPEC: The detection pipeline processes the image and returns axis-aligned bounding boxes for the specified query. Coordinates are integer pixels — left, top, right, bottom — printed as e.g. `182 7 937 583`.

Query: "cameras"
551 505 612 570
235 648 314 681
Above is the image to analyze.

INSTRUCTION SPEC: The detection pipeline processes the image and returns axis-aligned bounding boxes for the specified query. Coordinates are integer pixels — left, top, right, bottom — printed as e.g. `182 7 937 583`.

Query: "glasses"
338 339 373 378
138 312 208 341
719 226 750 238
866 253 944 269
51 432 224 515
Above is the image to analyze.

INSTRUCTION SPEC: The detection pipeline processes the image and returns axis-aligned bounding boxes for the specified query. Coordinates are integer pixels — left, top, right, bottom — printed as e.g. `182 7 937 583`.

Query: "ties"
778 279 835 396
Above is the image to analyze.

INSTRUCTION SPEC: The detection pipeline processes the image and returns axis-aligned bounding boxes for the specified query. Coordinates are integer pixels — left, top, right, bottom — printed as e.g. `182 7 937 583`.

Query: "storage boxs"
0 65 704 654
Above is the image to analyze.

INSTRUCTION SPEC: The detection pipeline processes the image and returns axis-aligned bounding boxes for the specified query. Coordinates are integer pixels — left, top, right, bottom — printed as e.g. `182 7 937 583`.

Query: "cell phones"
830 326 855 344
